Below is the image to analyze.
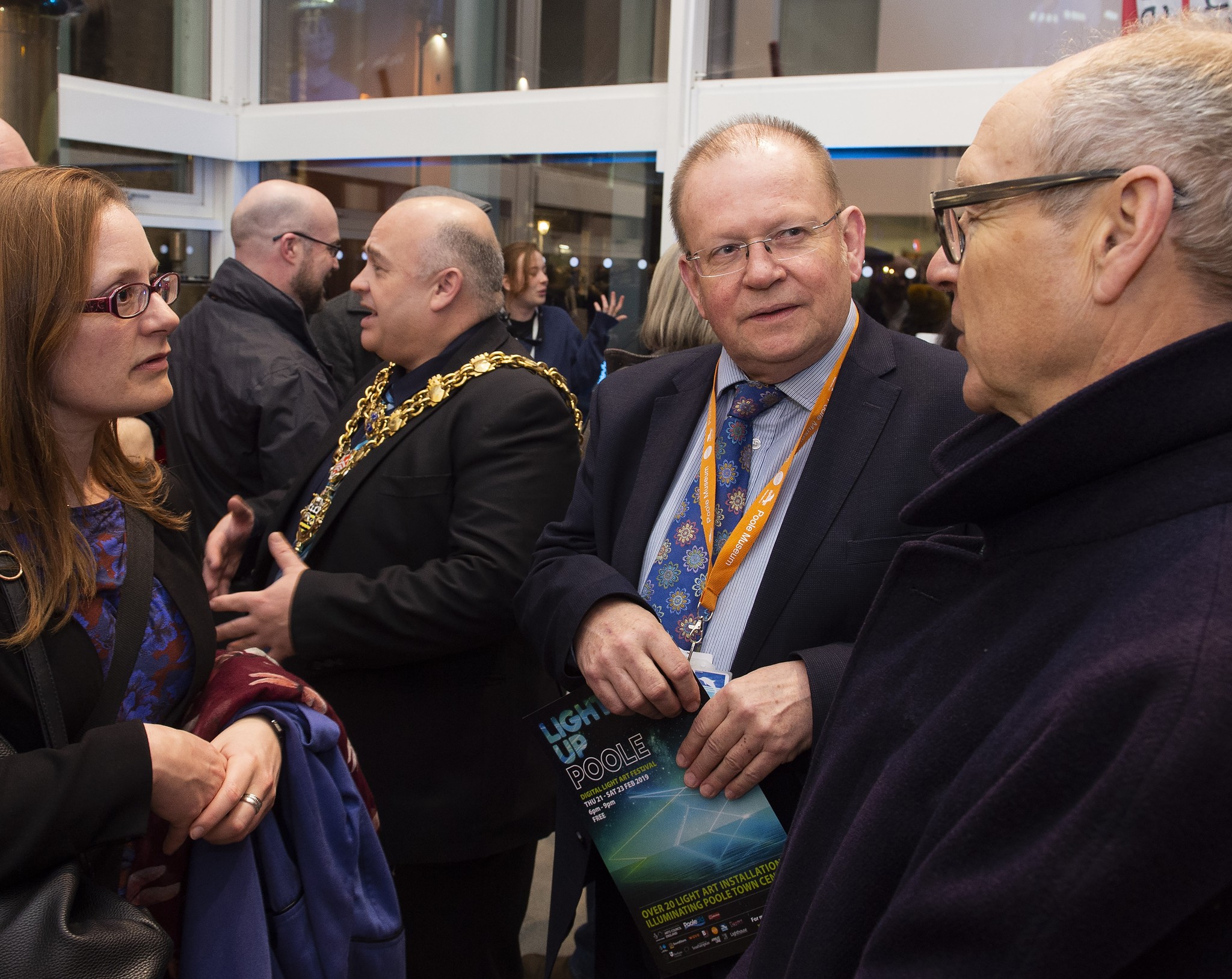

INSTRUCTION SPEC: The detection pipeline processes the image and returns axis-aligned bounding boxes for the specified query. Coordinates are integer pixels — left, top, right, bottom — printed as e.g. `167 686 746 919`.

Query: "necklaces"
294 351 585 556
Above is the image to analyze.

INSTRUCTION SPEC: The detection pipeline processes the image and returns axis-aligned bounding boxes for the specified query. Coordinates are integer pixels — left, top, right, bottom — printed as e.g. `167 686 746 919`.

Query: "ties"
640 381 785 651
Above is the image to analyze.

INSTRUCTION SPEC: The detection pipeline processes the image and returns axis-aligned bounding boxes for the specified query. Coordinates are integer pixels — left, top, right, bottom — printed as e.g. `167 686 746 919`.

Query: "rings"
240 793 262 815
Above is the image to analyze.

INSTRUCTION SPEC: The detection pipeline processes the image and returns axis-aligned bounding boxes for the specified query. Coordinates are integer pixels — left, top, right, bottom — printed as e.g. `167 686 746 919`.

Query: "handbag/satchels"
0 735 170 979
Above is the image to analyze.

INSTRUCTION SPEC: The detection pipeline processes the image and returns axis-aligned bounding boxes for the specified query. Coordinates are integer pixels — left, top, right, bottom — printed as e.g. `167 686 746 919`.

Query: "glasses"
929 168 1186 264
685 211 840 277
83 272 179 318
273 231 340 257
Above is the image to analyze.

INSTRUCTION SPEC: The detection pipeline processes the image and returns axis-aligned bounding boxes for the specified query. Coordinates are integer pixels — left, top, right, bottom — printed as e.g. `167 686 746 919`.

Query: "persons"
1 20 1232 979
